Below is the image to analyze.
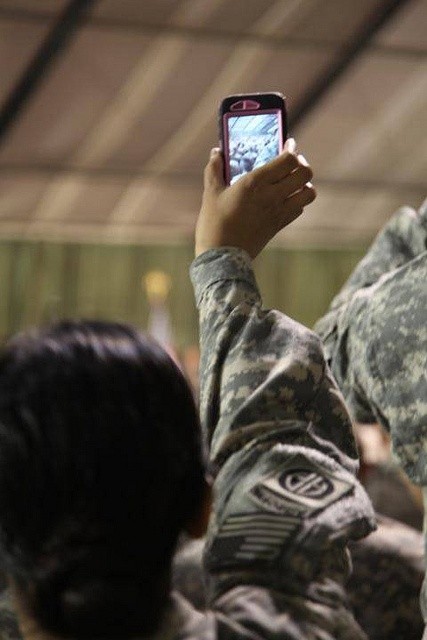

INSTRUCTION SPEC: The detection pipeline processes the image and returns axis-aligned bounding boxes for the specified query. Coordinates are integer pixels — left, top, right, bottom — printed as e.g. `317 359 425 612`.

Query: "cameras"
218 92 288 188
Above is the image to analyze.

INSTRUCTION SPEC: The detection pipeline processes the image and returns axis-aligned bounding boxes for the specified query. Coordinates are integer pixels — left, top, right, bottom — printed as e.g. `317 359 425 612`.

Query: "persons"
229 136 280 187
1 137 377 639
313 197 426 639
